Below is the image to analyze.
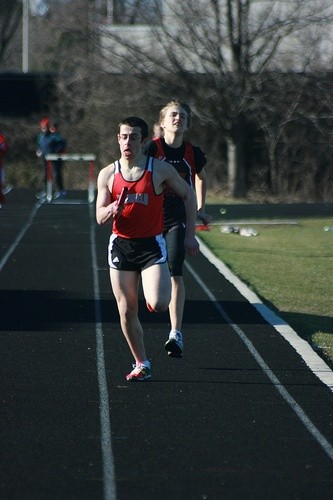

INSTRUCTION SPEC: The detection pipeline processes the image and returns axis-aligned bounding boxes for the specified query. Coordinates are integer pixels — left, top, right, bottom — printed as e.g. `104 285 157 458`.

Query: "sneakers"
147 303 156 313
126 362 151 381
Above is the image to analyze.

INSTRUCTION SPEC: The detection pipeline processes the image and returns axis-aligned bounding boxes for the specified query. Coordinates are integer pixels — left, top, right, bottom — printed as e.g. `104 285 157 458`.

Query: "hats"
40 119 49 125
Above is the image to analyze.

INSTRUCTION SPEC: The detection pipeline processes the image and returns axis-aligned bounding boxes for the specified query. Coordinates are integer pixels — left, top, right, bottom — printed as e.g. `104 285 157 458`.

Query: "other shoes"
54 191 68 200
34 193 46 199
164 333 184 358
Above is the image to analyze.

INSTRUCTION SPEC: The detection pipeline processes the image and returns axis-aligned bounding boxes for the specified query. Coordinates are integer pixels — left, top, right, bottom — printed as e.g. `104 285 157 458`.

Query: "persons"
96 117 198 382
36 118 51 198
46 121 67 200
151 119 164 140
144 102 211 357
0 133 9 209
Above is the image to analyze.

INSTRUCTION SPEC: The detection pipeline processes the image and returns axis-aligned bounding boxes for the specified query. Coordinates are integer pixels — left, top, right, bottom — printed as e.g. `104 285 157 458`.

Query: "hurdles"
44 154 96 204
